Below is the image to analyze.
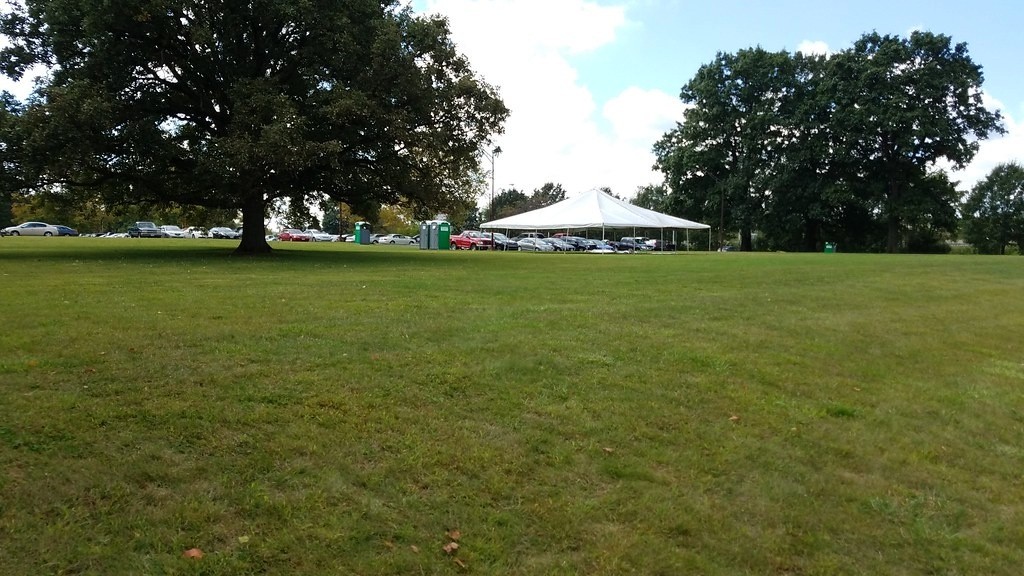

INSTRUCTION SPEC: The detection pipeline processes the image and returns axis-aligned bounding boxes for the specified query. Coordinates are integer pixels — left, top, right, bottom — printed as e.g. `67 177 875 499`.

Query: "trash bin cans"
418 219 431 250
428 220 451 250
823 241 838 254
353 221 372 245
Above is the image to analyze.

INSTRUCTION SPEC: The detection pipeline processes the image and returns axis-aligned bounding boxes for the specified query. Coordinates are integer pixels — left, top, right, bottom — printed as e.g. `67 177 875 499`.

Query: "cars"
1 221 59 237
448 229 676 253
49 224 79 238
80 220 241 240
264 227 420 247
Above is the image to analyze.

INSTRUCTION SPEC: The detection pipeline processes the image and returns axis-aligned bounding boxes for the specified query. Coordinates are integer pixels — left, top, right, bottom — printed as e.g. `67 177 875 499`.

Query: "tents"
479 187 711 254
